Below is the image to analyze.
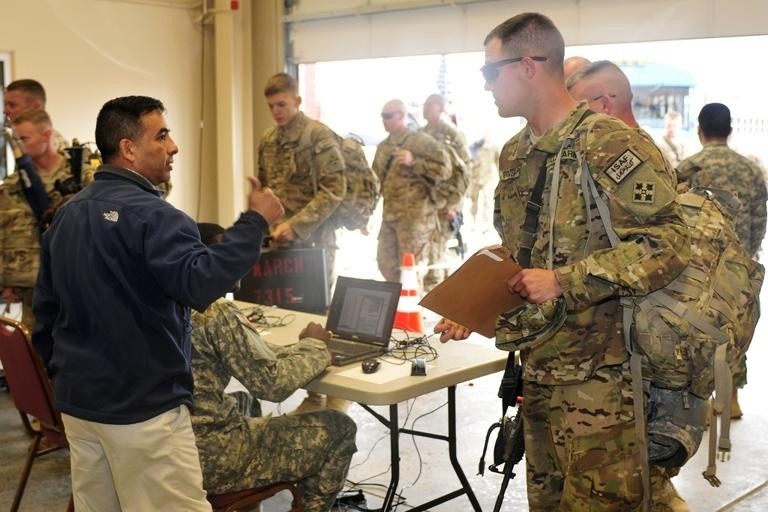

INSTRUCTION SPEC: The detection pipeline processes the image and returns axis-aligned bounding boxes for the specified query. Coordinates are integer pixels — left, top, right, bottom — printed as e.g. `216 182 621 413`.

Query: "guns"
489 352 523 511
54 146 84 193
448 208 466 259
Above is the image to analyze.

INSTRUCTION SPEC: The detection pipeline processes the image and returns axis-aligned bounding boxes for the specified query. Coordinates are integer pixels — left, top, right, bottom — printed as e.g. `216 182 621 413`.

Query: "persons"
658 108 685 169
190 222 358 512
256 72 348 302
0 107 94 454
564 56 591 79
676 102 767 421
3 78 71 156
471 128 500 232
417 93 471 286
362 99 451 291
434 11 693 511
567 59 676 181
32 95 285 512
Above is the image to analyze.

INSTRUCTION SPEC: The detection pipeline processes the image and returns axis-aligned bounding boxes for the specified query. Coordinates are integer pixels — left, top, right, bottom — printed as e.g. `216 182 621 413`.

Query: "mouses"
361 358 383 373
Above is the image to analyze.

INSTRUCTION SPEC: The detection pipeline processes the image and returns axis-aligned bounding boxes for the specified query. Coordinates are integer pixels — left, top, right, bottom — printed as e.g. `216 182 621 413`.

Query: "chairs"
207 475 304 512
0 316 77 511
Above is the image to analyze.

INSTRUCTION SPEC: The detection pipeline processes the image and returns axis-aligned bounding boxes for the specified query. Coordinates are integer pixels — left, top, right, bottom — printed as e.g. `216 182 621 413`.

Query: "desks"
230 301 519 512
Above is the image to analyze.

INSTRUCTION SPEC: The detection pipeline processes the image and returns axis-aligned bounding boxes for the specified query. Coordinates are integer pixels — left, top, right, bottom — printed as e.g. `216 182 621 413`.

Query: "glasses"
381 110 401 119
481 55 546 81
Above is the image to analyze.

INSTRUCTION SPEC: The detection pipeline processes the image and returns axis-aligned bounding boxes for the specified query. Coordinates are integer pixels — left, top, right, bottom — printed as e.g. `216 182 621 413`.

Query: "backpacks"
301 121 381 235
405 131 470 204
575 127 765 506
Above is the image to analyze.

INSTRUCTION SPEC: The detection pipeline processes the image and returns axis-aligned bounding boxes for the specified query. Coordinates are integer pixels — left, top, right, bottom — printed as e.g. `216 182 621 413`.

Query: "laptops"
280 276 403 368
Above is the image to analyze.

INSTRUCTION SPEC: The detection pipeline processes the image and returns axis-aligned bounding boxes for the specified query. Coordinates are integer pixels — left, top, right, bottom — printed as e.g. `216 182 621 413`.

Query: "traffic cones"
393 251 425 332
